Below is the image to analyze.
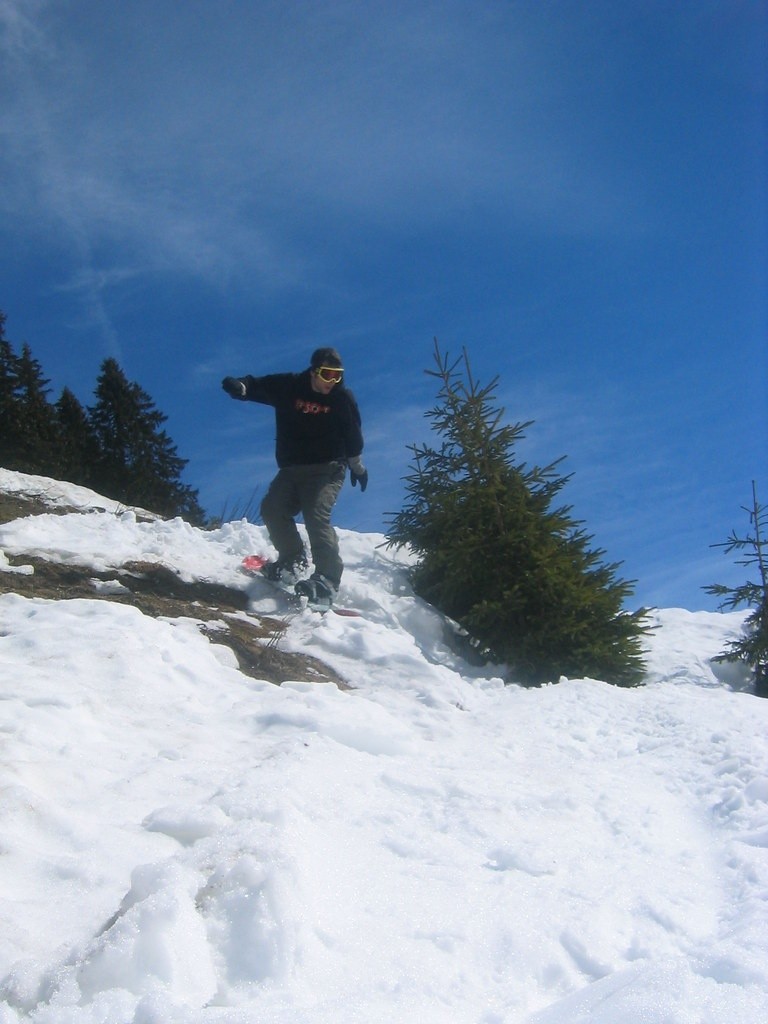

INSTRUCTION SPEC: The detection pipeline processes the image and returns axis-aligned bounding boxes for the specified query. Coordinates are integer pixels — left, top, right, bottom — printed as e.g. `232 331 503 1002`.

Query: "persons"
223 348 367 601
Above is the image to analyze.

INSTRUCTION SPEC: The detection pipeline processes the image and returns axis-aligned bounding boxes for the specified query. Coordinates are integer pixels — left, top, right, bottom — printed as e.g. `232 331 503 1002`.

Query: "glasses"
313 367 344 383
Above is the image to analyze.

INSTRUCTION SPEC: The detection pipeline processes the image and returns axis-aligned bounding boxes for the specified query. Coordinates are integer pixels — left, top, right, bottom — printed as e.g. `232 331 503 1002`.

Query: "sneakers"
294 575 337 606
264 557 308 580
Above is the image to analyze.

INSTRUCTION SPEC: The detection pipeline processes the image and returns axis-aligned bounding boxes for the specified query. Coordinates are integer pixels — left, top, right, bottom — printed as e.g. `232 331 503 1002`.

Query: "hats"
310 348 341 368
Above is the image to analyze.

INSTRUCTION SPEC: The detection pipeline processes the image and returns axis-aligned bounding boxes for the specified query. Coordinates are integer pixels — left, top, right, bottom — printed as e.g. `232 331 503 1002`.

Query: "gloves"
348 457 368 492
221 375 247 400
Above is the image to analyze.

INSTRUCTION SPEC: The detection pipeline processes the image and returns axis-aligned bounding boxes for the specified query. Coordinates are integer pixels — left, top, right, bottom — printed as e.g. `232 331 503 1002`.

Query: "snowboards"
241 554 360 617
